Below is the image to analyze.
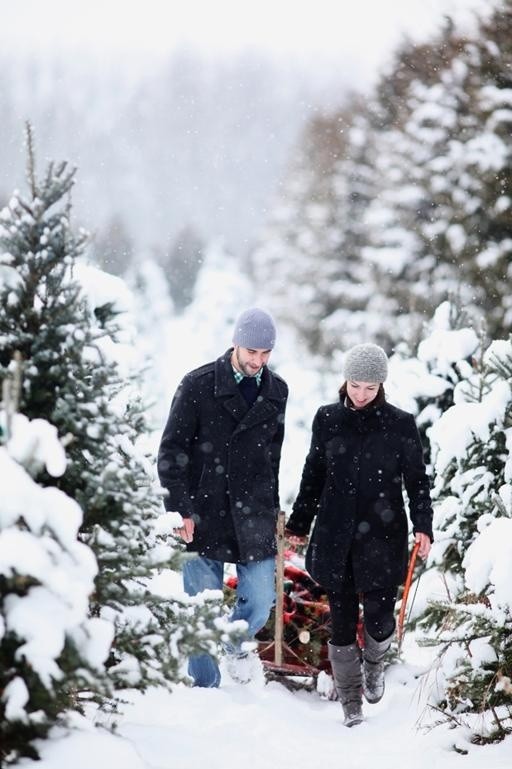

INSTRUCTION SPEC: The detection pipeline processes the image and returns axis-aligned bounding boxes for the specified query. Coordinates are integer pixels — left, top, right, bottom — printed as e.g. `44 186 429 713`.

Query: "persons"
157 303 289 689
281 342 434 729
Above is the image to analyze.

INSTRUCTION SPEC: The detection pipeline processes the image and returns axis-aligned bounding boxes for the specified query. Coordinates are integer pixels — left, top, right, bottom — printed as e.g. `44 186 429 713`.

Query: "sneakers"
229 656 253 685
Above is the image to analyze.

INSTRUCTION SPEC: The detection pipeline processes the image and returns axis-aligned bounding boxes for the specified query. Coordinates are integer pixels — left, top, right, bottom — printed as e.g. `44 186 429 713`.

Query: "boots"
327 619 394 727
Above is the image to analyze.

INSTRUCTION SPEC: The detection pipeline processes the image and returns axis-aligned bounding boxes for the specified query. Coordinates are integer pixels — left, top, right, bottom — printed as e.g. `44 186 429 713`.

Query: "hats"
343 343 389 383
232 309 276 349
